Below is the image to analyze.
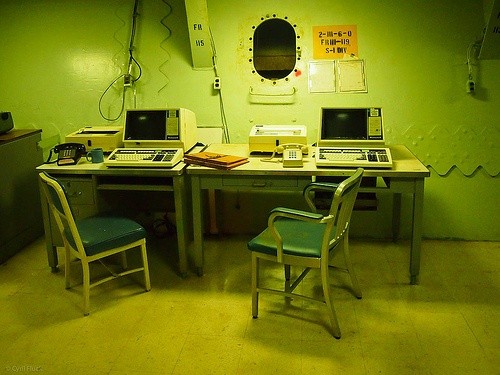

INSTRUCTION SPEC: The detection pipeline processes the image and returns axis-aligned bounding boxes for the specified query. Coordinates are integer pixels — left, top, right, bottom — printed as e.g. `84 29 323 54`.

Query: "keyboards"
316 146 393 167
105 147 183 167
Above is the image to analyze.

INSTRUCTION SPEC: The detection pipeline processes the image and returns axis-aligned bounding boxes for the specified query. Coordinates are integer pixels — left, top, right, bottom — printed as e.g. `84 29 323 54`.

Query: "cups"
86 148 104 163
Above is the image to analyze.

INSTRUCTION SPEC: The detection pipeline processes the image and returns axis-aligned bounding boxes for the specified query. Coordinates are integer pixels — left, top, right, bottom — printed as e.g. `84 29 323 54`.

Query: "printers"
251 124 308 154
65 126 123 152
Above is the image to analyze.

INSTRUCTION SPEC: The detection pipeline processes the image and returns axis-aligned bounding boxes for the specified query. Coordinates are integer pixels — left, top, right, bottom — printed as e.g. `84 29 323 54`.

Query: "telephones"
277 143 308 167
54 143 85 166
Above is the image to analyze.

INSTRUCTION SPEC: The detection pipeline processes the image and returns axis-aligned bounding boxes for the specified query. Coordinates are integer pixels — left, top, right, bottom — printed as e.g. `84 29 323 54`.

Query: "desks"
186 142 430 285
35 143 209 278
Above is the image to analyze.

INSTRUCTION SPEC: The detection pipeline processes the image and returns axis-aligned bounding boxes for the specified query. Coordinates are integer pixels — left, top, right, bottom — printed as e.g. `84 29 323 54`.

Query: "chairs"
37 171 151 317
247 166 364 339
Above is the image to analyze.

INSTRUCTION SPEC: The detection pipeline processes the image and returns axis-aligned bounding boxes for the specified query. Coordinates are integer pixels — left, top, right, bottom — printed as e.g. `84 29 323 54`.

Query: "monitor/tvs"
123 108 196 151
318 107 385 144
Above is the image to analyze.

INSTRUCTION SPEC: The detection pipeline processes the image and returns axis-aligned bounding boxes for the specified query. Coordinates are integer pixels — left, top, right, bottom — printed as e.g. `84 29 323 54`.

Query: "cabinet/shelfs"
0 129 47 266
